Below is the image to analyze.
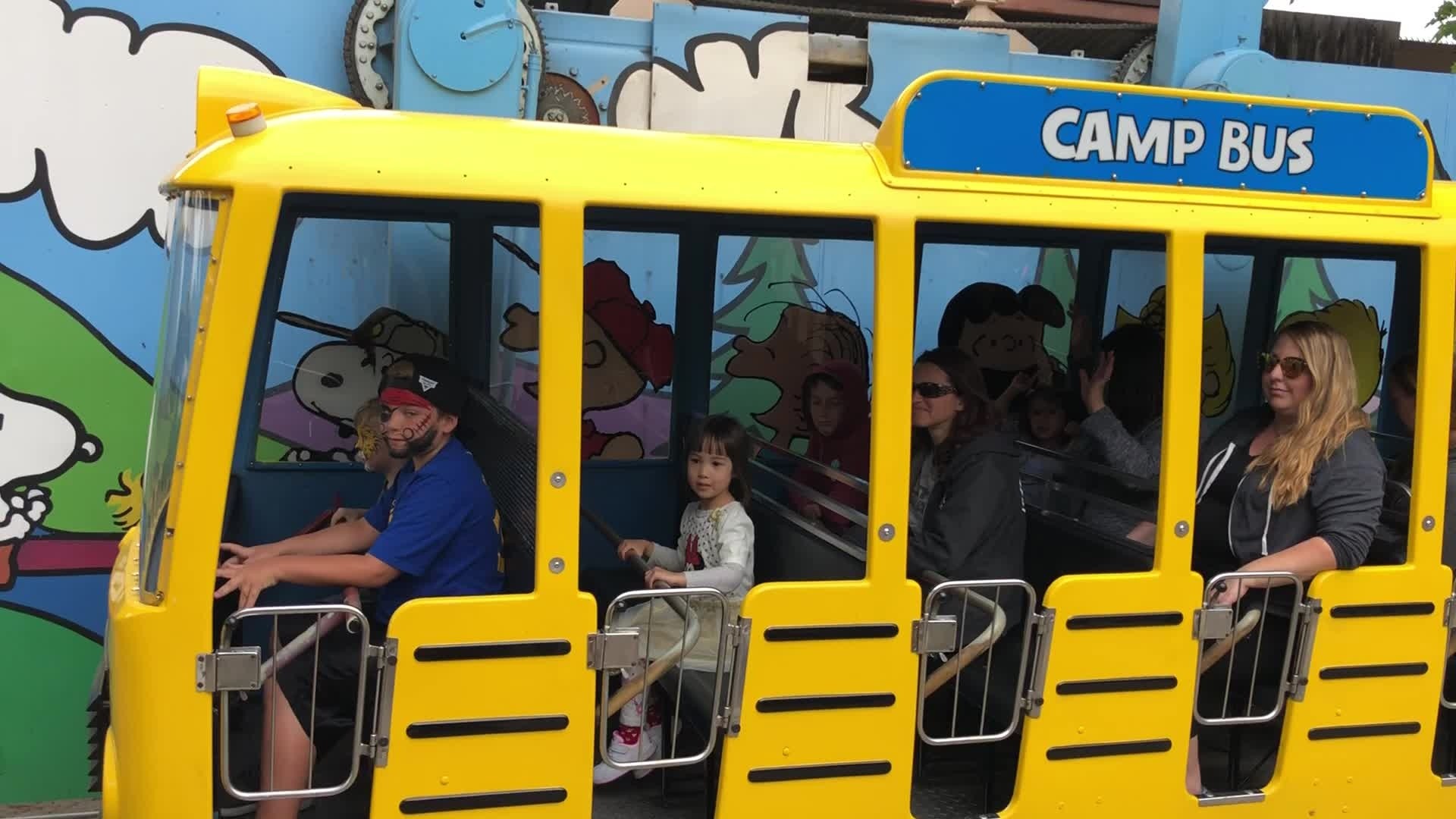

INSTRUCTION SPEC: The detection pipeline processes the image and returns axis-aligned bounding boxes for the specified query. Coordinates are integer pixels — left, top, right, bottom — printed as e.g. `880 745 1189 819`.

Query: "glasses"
1259 352 1306 378
912 381 957 397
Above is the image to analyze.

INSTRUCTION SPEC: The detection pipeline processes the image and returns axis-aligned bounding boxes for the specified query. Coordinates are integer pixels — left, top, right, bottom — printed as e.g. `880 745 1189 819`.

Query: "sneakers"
594 729 656 785
633 715 682 780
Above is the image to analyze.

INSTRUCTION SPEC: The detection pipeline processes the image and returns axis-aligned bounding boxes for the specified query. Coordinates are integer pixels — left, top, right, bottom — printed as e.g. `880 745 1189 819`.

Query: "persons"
793 360 872 527
906 320 1385 794
1390 342 1456 660
214 359 508 819
591 413 754 786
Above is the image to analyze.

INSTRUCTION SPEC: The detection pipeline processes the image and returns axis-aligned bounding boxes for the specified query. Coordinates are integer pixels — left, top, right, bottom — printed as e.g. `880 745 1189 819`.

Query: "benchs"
1239 478 1412 731
338 380 556 761
919 502 1166 741
581 475 892 768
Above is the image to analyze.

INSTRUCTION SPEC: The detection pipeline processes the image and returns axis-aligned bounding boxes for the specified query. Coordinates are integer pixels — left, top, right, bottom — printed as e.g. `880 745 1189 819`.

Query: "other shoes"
220 777 313 818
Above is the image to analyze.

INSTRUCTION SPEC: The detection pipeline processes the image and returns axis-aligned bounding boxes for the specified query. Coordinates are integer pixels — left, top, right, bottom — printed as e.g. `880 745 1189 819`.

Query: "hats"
379 353 469 416
